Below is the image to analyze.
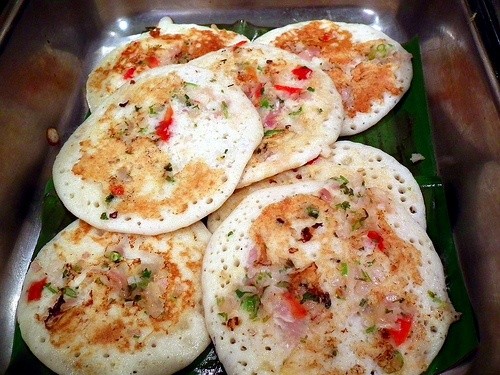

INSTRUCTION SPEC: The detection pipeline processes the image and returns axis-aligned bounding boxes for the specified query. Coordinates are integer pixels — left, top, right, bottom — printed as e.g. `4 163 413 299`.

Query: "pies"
17 16 463 375
